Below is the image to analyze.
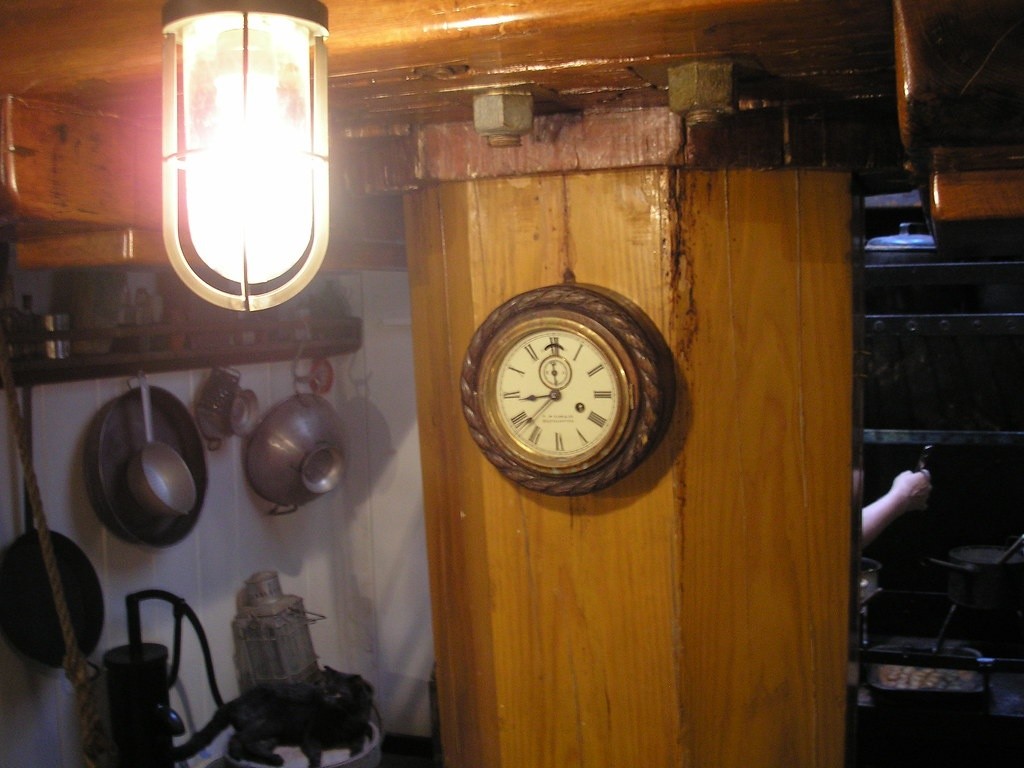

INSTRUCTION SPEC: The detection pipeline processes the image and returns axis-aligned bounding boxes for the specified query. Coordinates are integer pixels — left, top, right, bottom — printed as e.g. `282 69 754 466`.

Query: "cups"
43 312 71 359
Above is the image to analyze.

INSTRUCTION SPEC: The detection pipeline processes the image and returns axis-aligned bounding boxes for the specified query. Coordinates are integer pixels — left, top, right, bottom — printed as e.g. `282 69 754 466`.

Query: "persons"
862 468 932 543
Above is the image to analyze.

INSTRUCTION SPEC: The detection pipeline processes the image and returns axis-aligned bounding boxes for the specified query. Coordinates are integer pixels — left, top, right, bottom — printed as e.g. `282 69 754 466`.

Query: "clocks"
480 309 643 483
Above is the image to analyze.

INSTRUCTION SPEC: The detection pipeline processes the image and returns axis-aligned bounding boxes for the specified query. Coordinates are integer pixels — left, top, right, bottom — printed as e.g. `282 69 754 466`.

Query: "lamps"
159 0 336 309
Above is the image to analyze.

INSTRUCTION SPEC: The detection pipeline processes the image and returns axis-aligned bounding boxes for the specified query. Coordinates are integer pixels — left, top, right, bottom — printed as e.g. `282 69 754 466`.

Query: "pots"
928 536 1024 609
244 374 351 517
83 384 208 549
118 372 197 538
0 471 105 684
